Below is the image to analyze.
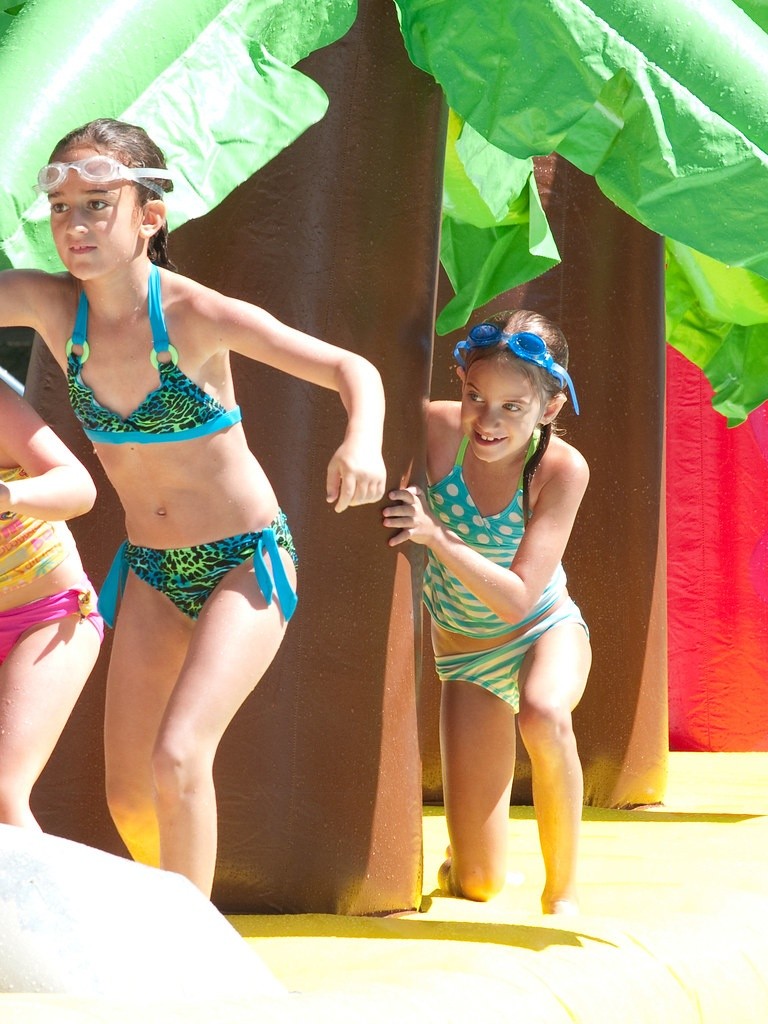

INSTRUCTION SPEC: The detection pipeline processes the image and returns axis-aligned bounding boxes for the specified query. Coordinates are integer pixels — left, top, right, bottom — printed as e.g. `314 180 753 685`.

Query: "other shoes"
437 842 454 893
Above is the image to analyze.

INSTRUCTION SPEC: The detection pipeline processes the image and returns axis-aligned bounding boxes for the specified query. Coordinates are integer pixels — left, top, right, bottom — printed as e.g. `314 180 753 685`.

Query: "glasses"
453 322 587 416
34 156 178 200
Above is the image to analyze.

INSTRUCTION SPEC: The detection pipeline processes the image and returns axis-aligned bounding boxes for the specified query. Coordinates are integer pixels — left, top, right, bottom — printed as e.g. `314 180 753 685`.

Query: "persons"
0 377 110 838
0 117 388 910
383 308 594 919
